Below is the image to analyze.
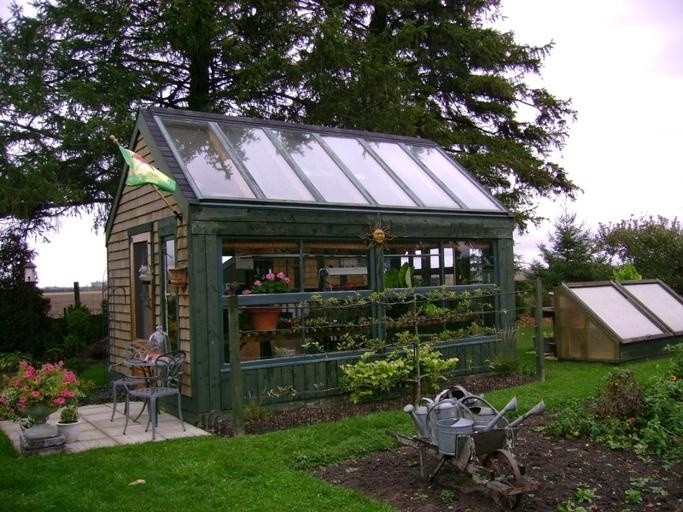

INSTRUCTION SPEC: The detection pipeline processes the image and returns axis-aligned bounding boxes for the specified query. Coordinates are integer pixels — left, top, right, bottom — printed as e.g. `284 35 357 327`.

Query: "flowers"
241 271 289 294
0 358 80 423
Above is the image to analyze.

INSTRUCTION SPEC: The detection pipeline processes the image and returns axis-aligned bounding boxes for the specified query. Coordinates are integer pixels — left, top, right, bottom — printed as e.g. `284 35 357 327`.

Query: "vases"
14 403 61 439
248 307 279 331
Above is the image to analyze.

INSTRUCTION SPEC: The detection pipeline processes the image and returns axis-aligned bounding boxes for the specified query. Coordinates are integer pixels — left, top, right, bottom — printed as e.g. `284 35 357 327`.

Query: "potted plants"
56 408 80 445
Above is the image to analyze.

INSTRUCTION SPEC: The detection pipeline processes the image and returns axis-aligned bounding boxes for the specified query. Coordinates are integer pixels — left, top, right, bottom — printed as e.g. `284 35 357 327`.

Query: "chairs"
108 340 185 440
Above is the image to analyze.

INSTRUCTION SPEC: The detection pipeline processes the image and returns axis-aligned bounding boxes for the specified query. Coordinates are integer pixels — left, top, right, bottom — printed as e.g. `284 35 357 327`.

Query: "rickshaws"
382 423 540 507
